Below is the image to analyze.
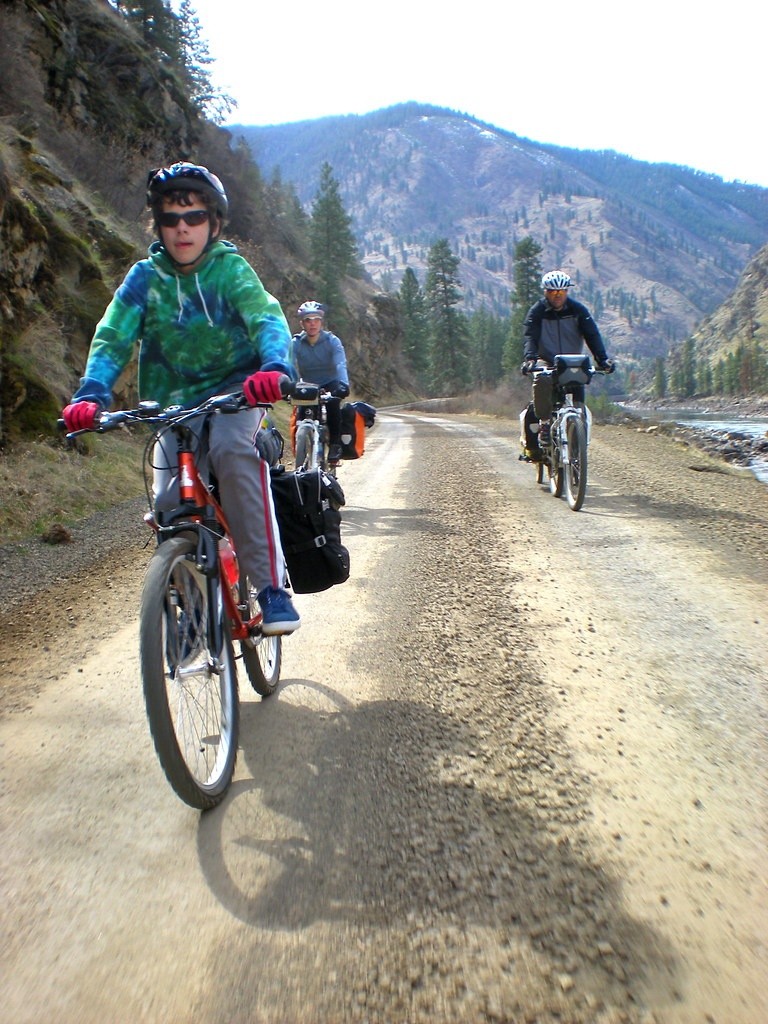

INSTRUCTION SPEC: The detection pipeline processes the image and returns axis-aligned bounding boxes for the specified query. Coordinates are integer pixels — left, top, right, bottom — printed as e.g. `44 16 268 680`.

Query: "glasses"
158 210 209 227
546 289 568 295
305 318 319 323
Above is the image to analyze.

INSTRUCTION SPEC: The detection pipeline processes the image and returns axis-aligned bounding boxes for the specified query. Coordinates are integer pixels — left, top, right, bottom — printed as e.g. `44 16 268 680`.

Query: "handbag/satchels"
339 402 365 460
268 465 349 595
518 402 543 462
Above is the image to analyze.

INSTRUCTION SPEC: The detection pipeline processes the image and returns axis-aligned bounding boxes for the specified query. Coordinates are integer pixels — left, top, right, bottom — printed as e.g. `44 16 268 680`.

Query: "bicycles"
282 386 336 475
57 384 317 810
518 355 612 511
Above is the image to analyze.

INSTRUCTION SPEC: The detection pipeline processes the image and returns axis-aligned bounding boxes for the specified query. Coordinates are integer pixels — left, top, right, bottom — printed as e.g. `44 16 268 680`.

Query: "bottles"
214 520 240 588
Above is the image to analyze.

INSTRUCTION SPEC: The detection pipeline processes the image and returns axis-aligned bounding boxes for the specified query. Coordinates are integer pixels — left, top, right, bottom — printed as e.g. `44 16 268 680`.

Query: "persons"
520 272 616 460
60 163 302 634
290 301 352 462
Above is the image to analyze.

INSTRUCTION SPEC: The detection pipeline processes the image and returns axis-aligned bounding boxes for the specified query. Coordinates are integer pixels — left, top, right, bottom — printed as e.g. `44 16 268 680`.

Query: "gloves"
62 401 103 433
598 358 615 374
243 372 289 406
331 381 350 399
519 359 535 376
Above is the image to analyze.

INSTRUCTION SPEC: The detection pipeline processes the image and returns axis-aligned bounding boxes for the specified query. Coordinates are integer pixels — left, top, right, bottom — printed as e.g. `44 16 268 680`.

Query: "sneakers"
170 609 202 666
256 584 300 634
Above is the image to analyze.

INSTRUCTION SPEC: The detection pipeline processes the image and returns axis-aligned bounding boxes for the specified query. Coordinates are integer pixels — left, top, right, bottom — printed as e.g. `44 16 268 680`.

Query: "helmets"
541 271 571 289
297 301 325 319
147 161 229 209
